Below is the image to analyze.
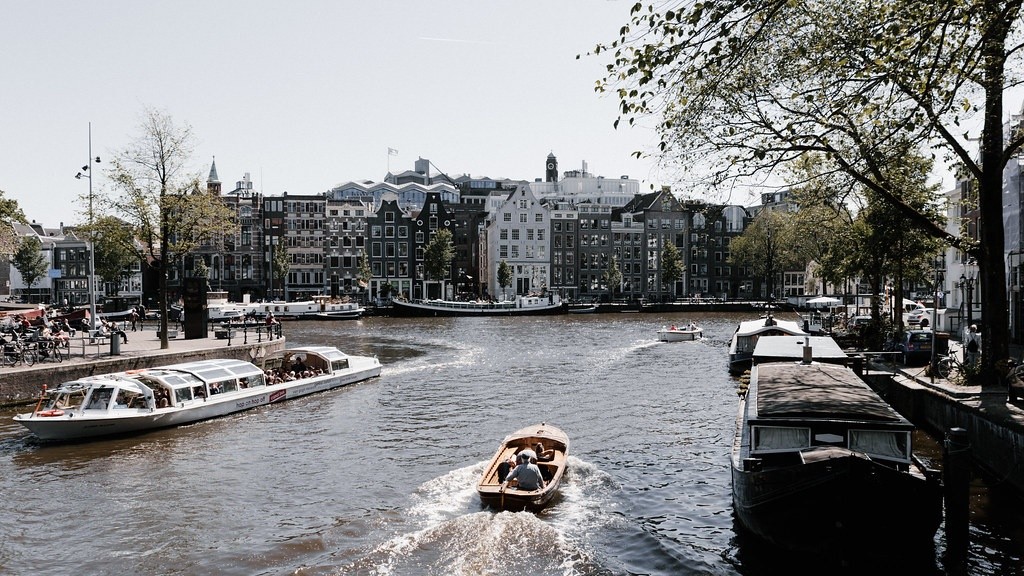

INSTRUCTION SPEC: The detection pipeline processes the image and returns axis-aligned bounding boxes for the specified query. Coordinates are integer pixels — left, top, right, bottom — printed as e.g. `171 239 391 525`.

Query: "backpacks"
968 332 978 353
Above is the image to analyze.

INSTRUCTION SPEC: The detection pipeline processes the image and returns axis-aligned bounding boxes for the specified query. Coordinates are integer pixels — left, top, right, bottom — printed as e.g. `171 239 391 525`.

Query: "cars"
908 308 940 326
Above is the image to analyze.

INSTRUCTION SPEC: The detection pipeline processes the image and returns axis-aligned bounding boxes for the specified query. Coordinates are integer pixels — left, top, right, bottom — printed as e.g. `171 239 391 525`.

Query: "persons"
266 312 280 341
670 324 676 330
690 321 698 330
499 452 546 493
963 324 982 386
1 305 186 362
685 324 693 331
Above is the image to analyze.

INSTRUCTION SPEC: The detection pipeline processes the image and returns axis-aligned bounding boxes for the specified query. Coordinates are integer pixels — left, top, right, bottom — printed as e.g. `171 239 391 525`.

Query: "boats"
14 345 382 440
390 289 570 317
209 295 365 324
656 319 703 342
726 308 943 539
477 421 570 513
